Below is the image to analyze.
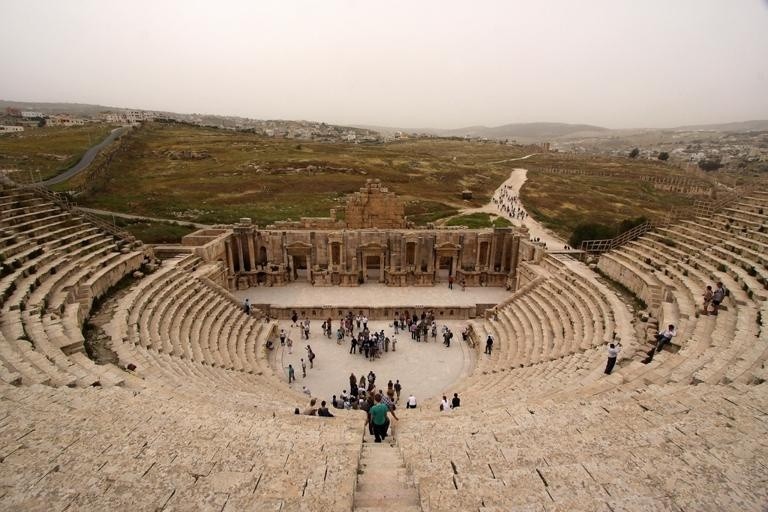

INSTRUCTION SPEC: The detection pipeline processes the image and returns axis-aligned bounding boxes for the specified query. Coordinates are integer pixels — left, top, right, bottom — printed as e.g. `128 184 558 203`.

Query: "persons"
448 272 454 290
243 296 498 444
707 281 724 315
654 324 676 352
701 285 713 315
461 278 465 289
604 343 623 375
492 182 572 250
